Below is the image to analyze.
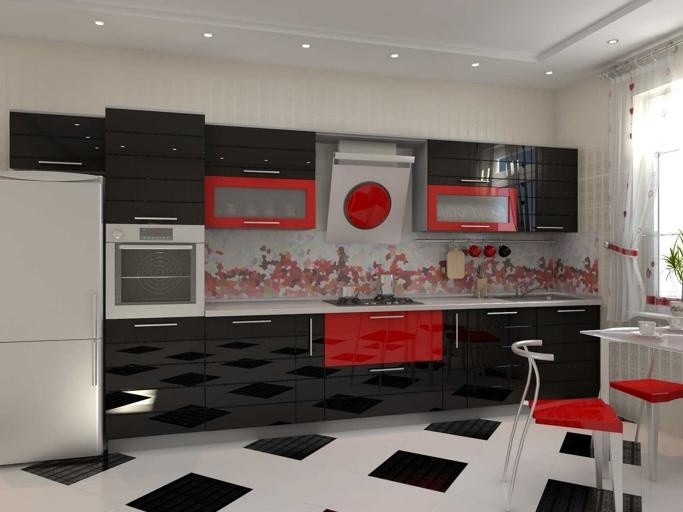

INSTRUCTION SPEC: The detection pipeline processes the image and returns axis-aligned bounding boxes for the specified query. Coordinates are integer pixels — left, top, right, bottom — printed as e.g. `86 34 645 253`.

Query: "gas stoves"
323 296 424 307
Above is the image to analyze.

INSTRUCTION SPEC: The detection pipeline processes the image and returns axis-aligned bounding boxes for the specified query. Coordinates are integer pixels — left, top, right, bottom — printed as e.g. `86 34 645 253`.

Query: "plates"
633 330 662 338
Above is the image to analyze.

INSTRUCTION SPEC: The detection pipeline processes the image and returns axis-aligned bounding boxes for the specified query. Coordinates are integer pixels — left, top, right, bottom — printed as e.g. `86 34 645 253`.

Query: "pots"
337 281 359 299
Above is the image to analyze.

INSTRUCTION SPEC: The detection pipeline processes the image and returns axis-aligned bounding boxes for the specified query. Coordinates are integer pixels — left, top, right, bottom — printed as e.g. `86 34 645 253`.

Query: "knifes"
476 261 485 279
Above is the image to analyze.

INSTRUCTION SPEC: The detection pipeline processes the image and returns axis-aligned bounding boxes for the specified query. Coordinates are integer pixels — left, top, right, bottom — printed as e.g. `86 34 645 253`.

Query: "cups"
469 245 481 258
500 245 512 258
224 204 300 218
483 245 497 257
638 319 657 334
376 273 396 296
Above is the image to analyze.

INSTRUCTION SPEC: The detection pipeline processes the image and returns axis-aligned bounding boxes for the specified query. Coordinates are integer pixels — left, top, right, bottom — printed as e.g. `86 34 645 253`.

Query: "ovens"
104 223 204 321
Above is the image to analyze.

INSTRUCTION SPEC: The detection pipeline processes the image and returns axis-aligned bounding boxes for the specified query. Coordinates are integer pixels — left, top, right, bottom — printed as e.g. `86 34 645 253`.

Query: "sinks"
492 293 580 302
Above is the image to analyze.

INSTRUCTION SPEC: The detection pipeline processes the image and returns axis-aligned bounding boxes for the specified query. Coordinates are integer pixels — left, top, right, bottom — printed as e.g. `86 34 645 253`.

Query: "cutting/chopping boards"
444 246 464 279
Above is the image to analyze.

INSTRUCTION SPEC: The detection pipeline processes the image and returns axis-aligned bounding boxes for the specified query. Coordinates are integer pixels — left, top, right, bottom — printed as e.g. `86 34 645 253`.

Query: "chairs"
501 339 623 512
607 346 681 473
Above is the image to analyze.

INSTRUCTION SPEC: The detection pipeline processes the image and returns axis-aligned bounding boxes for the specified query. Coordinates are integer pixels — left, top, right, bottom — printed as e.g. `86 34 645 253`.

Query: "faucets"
515 279 549 297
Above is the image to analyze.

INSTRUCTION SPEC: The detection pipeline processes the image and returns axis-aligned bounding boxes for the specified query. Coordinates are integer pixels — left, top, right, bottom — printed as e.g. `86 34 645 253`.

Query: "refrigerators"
0 171 106 467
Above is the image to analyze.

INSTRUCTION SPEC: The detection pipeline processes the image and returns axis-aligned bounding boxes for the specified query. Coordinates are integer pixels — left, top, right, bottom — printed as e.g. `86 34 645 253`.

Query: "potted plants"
663 231 683 317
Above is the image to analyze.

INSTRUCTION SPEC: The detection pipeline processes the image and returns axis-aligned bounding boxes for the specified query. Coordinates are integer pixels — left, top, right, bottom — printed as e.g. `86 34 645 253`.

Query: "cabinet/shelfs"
524 145 578 234
205 317 297 431
103 316 206 440
444 308 536 410
297 309 444 423
204 124 316 230
9 110 106 175
412 139 523 233
536 304 602 401
104 108 206 225
